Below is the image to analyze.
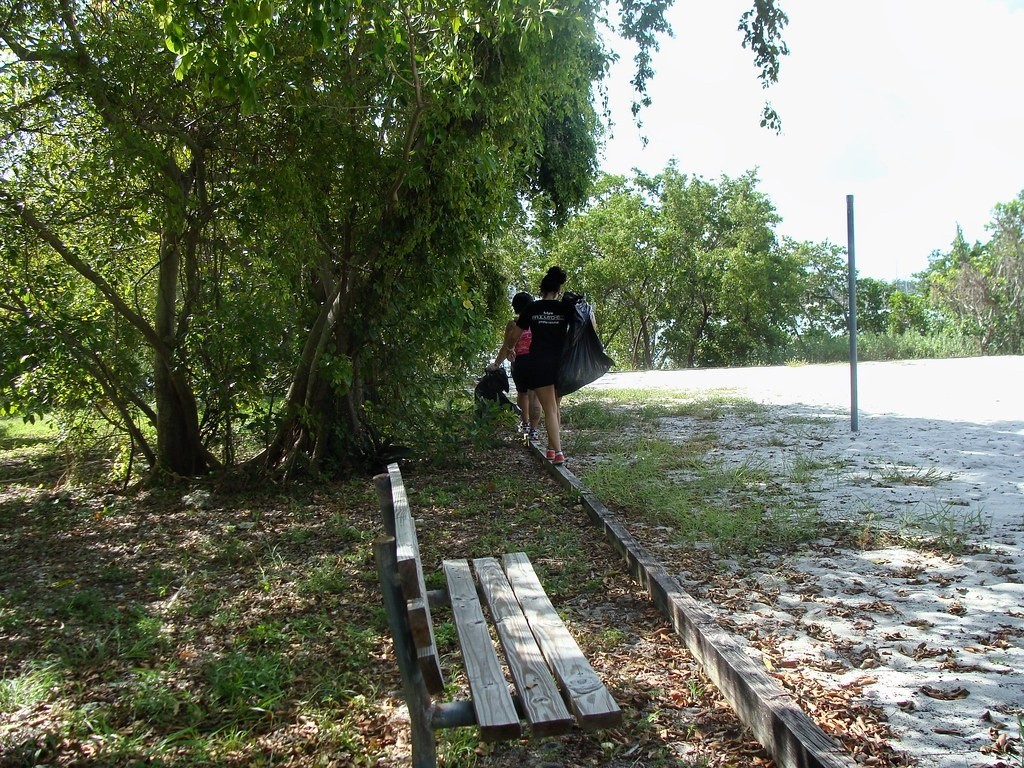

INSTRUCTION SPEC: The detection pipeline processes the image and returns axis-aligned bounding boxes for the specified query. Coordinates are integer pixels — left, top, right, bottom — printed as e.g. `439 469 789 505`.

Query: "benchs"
373 463 622 768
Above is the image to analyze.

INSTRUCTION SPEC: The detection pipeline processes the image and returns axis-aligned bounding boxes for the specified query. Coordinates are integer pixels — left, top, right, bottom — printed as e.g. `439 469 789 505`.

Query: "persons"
485 266 584 464
504 292 543 440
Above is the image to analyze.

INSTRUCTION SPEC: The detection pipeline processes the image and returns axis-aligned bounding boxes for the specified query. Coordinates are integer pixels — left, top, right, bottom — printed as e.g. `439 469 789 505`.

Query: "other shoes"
546 450 555 460
552 454 565 464
529 429 540 441
522 422 531 433
